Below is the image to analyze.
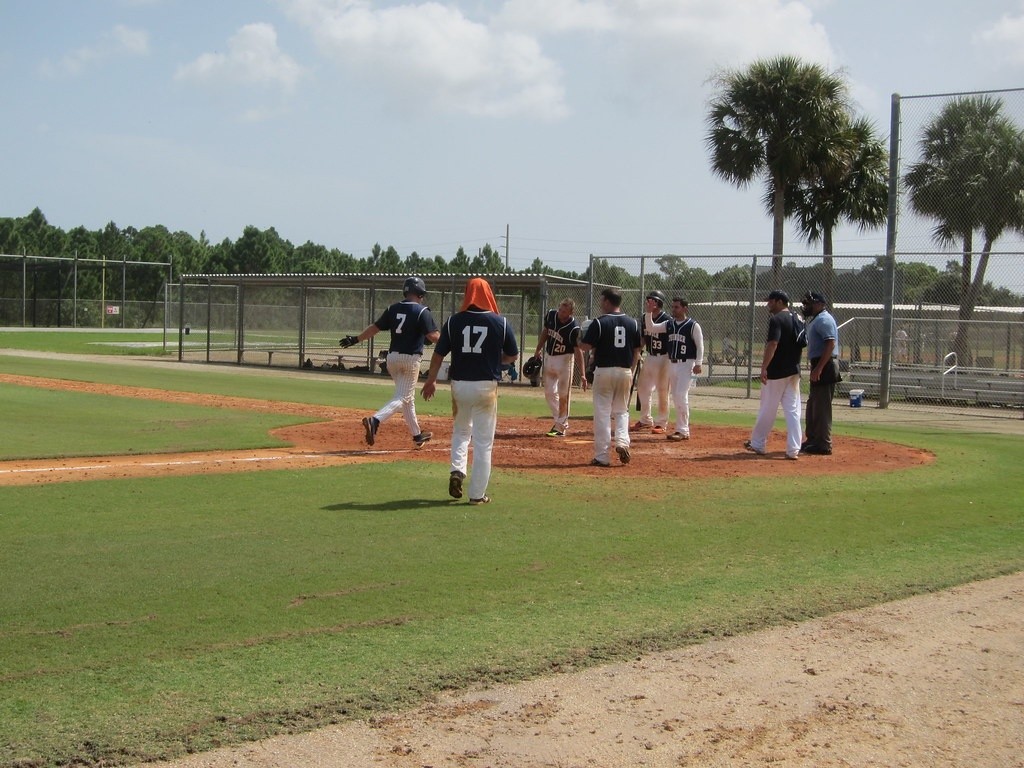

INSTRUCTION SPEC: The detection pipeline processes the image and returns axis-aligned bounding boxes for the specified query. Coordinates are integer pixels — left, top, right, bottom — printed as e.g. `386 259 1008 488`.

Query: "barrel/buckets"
437 363 449 380
849 389 861 407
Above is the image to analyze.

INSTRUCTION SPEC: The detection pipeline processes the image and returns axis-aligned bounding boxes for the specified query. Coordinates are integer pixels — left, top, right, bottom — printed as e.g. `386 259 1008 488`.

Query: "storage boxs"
848 389 865 408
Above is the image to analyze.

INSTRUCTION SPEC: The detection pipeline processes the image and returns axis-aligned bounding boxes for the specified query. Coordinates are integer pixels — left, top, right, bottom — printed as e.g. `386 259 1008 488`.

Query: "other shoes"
667 431 690 442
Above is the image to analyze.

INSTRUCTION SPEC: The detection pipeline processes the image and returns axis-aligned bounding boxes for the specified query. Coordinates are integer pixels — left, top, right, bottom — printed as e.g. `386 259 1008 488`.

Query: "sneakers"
629 421 653 431
448 472 464 499
652 426 667 434
616 446 631 464
784 452 799 460
743 440 765 455
362 417 379 446
546 425 566 437
413 430 433 449
590 457 611 467
799 442 832 456
469 494 491 505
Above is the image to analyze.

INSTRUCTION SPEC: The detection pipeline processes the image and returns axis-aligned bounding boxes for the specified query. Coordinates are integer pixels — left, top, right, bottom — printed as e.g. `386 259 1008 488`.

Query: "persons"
801 290 839 455
896 326 907 360
644 297 705 440
421 278 518 505
339 277 440 449
580 287 642 468
534 297 587 438
723 331 738 363
630 290 672 434
744 289 807 460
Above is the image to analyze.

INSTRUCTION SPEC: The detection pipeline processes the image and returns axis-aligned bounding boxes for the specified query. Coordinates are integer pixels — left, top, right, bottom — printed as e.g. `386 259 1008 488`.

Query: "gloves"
338 335 359 349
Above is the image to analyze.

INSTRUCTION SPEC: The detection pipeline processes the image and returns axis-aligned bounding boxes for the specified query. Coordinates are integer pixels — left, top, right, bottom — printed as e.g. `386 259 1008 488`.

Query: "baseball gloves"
523 357 542 378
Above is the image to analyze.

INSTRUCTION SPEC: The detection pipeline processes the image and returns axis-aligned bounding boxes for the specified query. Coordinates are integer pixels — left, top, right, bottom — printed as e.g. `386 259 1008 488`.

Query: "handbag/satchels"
792 312 807 347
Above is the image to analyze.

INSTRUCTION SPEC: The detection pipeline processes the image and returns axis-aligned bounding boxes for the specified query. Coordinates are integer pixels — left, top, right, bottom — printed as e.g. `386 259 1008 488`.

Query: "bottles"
691 372 697 388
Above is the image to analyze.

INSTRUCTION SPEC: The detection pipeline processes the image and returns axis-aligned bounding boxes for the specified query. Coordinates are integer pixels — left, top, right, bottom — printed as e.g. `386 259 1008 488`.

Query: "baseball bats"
628 349 645 411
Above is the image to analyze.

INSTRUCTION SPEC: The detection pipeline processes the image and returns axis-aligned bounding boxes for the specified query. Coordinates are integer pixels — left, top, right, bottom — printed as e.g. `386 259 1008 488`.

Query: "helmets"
402 277 428 297
646 290 666 305
523 356 543 380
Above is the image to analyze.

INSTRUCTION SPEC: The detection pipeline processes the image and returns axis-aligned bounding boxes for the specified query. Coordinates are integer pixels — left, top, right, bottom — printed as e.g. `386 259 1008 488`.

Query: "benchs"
837 363 1024 405
